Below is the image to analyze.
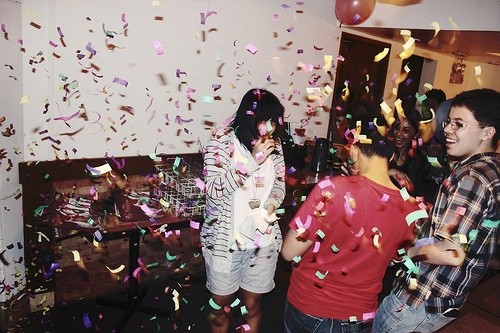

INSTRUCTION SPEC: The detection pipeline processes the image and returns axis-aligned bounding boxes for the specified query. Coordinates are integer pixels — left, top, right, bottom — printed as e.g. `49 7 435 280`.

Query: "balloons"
335 0 377 26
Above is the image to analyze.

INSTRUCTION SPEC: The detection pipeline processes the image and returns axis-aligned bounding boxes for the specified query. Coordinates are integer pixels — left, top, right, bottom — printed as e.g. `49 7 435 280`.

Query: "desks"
55 146 352 333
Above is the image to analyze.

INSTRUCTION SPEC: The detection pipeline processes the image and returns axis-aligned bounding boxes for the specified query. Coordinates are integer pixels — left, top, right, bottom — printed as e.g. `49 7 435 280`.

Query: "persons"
336 89 461 206
281 107 421 333
371 85 500 333
203 88 286 333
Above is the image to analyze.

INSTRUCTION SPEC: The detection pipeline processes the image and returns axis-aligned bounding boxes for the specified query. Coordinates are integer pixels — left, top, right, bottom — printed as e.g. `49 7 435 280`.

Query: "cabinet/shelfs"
152 159 206 217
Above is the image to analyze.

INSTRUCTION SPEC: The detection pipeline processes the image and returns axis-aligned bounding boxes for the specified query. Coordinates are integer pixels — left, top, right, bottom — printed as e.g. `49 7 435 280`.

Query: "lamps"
449 58 466 84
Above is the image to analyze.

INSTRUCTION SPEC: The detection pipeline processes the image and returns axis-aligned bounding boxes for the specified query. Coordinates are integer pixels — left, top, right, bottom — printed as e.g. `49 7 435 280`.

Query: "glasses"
441 120 490 130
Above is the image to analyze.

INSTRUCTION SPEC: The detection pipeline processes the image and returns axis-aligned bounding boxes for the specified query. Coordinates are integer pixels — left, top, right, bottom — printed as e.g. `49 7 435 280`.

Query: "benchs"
19 153 204 289
